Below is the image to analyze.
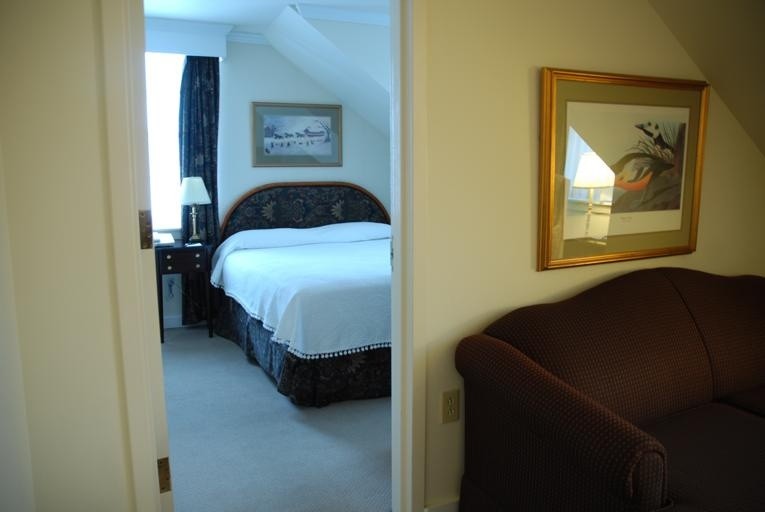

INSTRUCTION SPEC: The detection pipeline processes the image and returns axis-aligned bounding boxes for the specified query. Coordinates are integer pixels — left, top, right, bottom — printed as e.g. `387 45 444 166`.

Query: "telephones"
152 232 175 246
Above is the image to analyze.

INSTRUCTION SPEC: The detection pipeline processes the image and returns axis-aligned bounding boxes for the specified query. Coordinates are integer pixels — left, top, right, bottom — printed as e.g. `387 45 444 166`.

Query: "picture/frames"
251 101 344 168
535 66 710 272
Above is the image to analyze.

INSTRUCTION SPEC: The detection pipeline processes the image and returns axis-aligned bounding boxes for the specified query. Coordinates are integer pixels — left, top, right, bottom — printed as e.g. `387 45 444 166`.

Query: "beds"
217 181 391 408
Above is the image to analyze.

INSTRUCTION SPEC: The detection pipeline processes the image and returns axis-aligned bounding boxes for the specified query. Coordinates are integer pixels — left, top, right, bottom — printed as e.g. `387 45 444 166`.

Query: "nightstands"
155 239 213 344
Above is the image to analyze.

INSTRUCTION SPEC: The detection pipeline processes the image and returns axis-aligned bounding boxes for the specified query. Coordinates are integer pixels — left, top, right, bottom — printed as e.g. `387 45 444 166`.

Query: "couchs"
454 267 765 511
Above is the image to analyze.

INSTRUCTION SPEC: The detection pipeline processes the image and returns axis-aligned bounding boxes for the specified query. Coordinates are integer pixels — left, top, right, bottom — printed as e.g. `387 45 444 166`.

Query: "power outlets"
441 387 460 424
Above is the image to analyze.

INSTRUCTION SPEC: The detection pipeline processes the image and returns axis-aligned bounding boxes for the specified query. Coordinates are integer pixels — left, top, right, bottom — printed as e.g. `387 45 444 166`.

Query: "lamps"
177 176 212 245
